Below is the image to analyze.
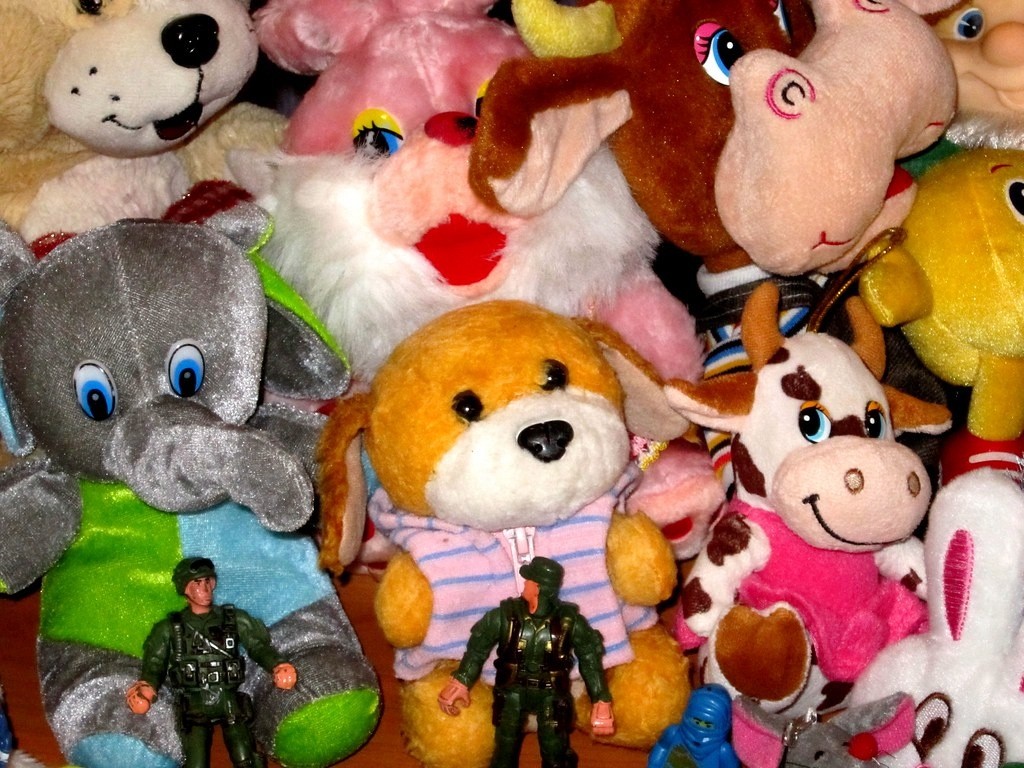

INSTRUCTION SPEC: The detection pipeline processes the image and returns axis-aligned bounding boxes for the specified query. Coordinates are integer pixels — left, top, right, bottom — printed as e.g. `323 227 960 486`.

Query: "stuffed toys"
0 0 1024 768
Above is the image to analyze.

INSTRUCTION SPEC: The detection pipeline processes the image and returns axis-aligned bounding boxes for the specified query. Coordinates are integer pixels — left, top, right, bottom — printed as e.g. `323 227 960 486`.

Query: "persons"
437 557 614 768
648 683 738 768
125 558 297 768
